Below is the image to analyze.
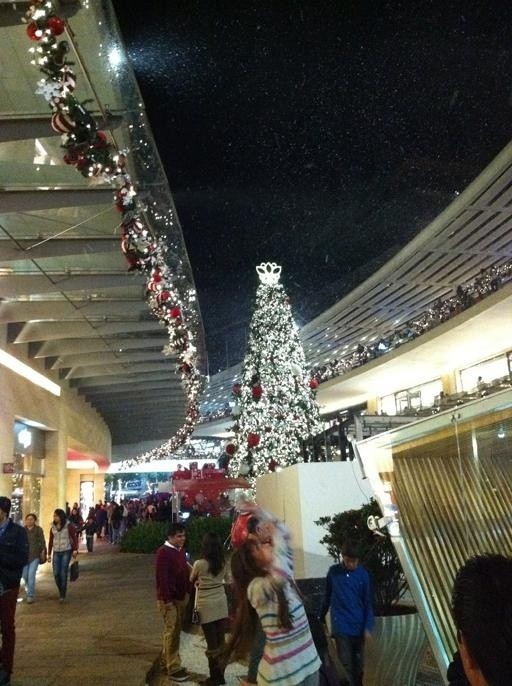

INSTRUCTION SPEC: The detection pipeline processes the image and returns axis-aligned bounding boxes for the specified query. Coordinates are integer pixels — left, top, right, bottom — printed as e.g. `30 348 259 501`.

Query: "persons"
0 497 29 686
82 517 95 552
22 514 46 604
95 494 172 544
48 509 78 604
453 554 512 686
70 508 83 539
86 507 95 519
73 503 78 509
66 502 70 517
189 530 228 686
318 539 374 686
206 493 322 686
155 523 191 681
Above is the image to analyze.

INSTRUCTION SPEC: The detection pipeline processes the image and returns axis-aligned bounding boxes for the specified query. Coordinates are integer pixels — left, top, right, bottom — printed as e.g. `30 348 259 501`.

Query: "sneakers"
168 669 191 682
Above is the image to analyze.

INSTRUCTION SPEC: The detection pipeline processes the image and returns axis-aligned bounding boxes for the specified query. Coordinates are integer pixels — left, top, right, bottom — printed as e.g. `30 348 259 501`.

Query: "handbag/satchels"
69 561 79 582
191 587 201 625
